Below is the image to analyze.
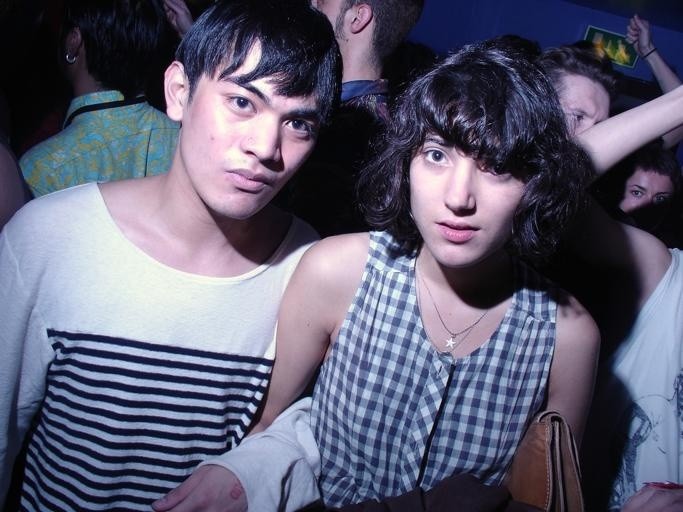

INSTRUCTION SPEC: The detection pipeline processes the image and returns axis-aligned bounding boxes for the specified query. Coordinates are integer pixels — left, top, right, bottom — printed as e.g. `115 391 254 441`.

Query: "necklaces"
415 260 491 354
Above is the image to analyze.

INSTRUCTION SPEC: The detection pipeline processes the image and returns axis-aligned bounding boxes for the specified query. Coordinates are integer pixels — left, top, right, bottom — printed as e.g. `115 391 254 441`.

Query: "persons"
0 2 346 512
0 1 681 246
239 35 602 512
568 79 682 512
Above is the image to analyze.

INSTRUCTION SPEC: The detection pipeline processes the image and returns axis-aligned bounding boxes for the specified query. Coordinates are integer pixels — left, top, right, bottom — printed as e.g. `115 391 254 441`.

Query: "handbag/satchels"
503 410 587 512
